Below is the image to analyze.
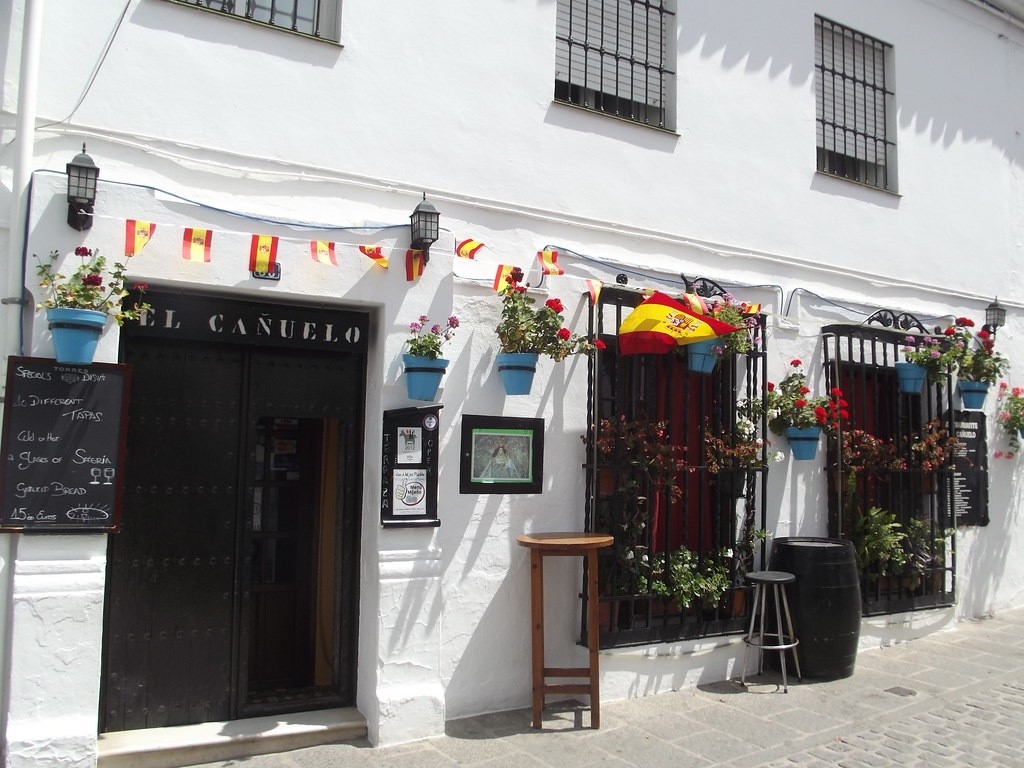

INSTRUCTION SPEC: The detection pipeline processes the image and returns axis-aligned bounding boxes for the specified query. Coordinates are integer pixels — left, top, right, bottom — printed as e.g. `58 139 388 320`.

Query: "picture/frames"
458 414 545 495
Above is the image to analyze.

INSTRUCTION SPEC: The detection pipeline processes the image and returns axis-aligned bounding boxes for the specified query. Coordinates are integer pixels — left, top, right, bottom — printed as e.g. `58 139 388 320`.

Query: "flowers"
944 317 1012 387
755 359 850 442
33 246 156 327
495 267 607 363
682 283 763 360
406 314 460 362
904 335 949 390
841 418 964 474
994 381 1024 459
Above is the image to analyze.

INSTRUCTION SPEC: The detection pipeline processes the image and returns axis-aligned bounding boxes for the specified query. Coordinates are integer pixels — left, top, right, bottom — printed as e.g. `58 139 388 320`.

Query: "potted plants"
594 446 682 633
842 469 956 594
643 529 766 618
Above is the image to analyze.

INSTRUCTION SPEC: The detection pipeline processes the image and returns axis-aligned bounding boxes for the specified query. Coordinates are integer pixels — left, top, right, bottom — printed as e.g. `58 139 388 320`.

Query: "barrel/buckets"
770 537 862 681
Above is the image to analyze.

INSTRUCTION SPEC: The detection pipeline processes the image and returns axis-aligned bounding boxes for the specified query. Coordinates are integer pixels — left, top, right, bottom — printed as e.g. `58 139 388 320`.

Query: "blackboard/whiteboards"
0 355 134 535
939 409 990 529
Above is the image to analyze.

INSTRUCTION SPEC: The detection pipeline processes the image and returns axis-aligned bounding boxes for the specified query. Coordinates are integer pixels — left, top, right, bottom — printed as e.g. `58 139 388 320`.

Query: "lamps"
984 296 1006 342
65 142 101 232
410 191 441 267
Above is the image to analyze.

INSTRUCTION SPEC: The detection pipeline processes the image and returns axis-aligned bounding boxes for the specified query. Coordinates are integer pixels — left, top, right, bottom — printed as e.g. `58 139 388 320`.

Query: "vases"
46 308 107 365
957 382 988 409
496 353 539 395
687 337 723 374
784 427 821 460
895 362 927 394
402 354 450 402
1019 425 1024 439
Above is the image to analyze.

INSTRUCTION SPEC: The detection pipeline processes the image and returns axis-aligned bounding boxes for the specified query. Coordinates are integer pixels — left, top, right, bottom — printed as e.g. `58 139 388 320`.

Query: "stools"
742 571 803 693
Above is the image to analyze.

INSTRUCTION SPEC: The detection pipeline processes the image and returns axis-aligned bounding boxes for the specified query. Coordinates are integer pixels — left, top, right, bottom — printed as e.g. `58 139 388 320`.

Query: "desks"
515 531 615 731
